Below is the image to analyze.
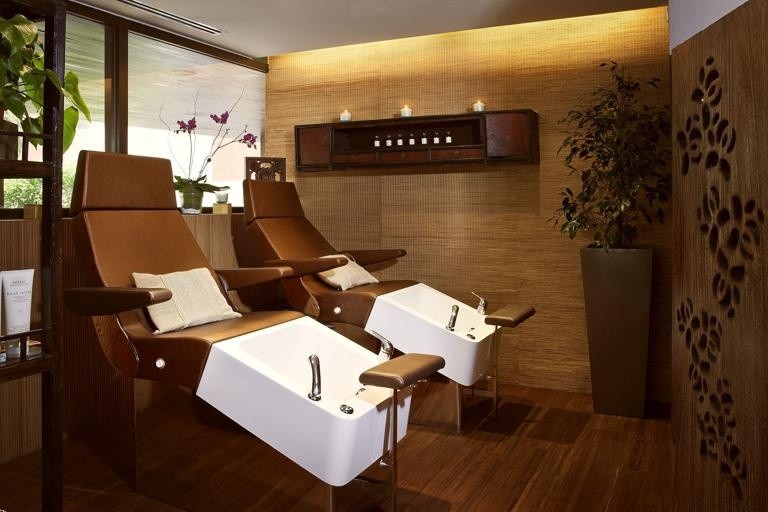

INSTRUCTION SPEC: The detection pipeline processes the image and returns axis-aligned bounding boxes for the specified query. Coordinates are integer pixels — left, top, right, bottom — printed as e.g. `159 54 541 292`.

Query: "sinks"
195 317 414 486
365 284 502 387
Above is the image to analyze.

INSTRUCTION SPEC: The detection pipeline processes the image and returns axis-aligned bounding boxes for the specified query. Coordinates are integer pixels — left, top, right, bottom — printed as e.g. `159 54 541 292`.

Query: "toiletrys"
2 269 35 359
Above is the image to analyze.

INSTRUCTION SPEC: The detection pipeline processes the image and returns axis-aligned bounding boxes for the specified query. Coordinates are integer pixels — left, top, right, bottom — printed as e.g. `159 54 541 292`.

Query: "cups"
181 193 203 214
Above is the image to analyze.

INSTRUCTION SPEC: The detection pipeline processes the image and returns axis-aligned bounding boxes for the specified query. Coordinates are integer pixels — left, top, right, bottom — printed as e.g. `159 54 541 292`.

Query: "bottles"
375 131 452 147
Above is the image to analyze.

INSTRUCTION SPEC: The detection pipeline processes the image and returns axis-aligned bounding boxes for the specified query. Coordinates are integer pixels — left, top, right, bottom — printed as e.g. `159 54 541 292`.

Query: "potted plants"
544 56 673 417
2 13 91 160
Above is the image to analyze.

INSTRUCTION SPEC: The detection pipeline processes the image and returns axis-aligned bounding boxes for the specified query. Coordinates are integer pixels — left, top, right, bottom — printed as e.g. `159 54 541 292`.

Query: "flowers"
156 82 261 196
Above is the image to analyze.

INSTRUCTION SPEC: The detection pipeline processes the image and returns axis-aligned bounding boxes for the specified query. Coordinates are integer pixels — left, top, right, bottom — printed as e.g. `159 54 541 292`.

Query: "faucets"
307 354 322 401
444 305 460 331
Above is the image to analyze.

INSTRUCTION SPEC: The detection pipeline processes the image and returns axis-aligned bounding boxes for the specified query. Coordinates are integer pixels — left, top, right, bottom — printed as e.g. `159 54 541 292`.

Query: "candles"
400 108 412 118
473 102 486 112
340 112 351 122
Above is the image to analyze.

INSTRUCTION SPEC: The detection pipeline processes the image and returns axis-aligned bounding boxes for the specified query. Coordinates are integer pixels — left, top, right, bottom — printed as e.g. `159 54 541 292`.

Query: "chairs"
241 177 536 435
61 151 447 511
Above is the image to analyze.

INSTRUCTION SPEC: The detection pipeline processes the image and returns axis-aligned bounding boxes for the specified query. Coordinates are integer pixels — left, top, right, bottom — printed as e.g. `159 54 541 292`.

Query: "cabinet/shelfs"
1 1 68 510
293 110 540 172
